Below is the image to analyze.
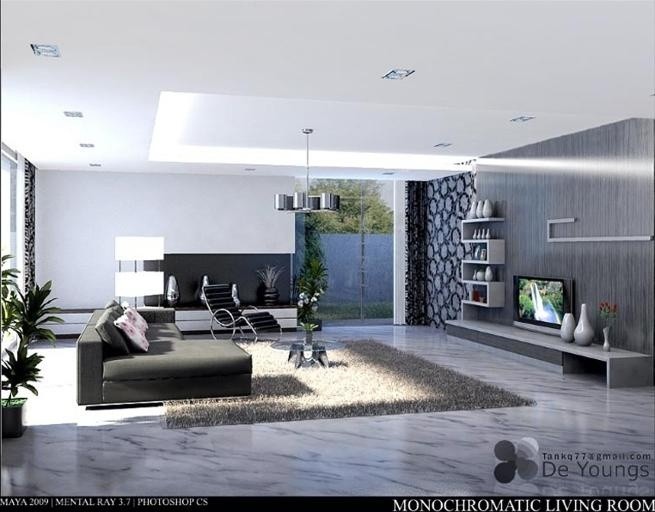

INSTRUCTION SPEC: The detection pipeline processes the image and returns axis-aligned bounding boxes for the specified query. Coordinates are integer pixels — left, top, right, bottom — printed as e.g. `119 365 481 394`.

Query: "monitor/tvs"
512 274 575 339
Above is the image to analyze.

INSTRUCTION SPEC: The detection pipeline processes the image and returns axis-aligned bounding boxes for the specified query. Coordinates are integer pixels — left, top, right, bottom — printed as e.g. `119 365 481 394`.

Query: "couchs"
76 300 252 406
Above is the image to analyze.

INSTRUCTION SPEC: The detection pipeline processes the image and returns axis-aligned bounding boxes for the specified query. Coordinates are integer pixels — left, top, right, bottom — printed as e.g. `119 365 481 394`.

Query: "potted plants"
255 264 288 306
0 250 65 438
289 322 330 371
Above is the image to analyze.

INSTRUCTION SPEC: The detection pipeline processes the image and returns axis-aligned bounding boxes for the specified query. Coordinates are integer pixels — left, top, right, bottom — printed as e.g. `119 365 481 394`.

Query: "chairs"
202 284 283 346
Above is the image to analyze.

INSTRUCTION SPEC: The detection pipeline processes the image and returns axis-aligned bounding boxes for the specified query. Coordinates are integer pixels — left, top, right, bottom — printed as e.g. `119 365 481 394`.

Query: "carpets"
160 337 538 429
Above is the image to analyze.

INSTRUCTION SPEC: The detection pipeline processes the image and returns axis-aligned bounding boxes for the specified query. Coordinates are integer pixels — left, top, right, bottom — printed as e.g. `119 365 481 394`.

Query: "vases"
602 327 610 352
301 319 323 331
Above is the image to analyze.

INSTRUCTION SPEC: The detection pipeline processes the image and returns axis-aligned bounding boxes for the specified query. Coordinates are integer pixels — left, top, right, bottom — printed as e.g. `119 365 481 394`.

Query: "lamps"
273 129 340 213
114 237 164 311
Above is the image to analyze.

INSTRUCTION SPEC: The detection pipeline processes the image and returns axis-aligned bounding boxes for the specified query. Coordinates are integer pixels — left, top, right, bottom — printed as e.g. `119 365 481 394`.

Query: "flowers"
600 302 617 327
295 237 330 322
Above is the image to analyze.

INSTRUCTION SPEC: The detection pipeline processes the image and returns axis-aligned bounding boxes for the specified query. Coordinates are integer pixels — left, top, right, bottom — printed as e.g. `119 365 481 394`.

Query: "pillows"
123 308 148 333
112 314 149 352
120 301 148 330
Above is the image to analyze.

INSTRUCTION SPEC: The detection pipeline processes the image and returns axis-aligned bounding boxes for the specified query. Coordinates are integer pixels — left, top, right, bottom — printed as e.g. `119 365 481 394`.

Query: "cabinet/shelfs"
460 217 505 325
444 318 653 389
37 306 299 339
